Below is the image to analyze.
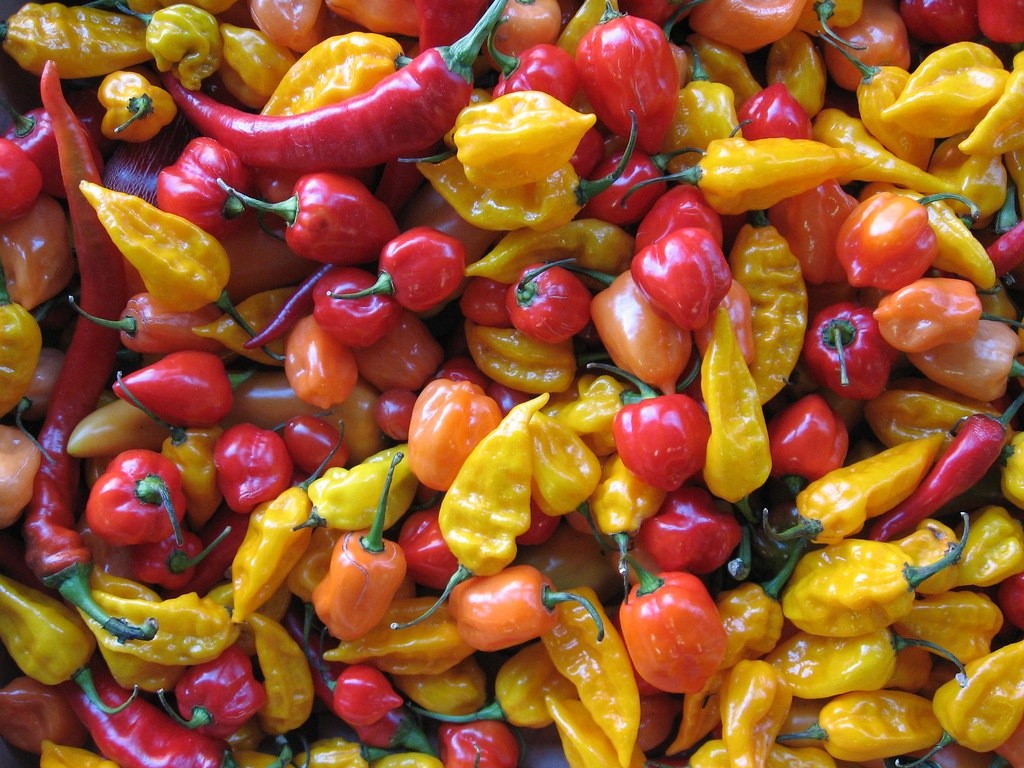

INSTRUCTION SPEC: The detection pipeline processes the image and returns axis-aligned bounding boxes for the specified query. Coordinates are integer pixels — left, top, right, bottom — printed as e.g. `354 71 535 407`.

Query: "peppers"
0 0 1024 768
